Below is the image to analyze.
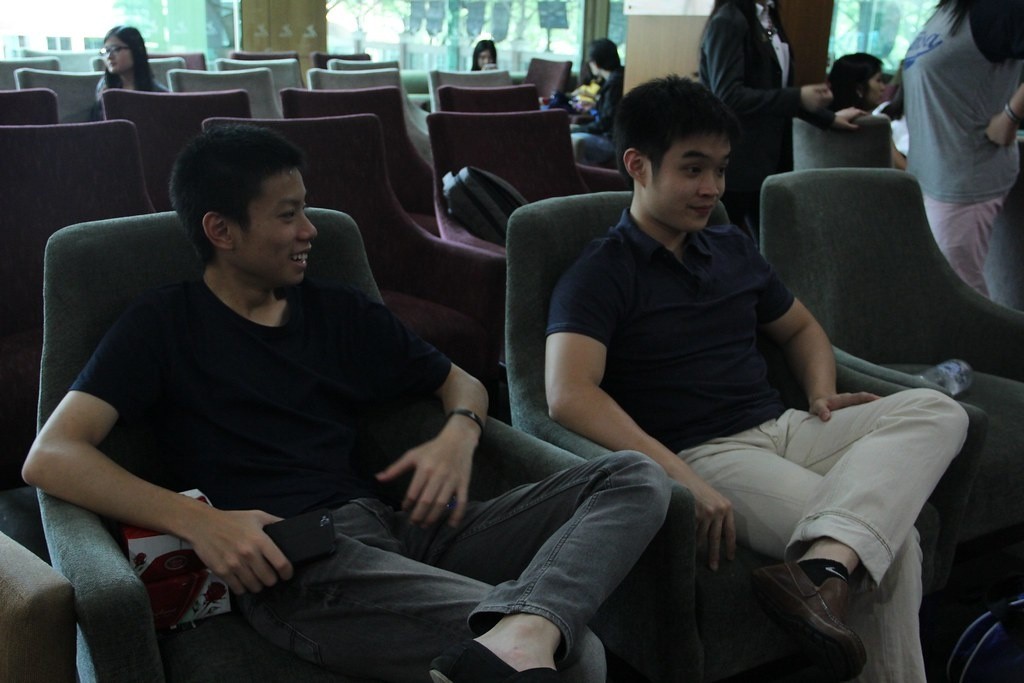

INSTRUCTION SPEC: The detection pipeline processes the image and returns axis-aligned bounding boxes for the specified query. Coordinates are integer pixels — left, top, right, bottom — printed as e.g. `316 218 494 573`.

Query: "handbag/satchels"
946 575 1024 683
441 166 528 246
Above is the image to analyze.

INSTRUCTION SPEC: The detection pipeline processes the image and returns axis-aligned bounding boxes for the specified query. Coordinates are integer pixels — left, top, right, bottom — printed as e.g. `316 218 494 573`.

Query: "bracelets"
1003 101 1021 124
445 408 486 440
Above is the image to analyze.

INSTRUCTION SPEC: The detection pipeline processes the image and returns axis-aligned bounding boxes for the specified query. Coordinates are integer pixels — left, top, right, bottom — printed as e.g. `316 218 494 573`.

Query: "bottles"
914 359 974 397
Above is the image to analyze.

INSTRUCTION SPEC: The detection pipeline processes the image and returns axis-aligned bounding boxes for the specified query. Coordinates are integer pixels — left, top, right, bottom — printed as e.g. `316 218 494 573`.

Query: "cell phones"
262 507 337 568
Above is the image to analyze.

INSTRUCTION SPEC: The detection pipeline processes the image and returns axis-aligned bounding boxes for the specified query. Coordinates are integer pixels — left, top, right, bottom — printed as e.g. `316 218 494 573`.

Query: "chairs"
1 46 1022 683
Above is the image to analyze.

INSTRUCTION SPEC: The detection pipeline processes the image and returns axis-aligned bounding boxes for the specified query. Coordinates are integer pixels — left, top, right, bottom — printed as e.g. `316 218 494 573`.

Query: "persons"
470 40 498 72
899 0 1024 298
540 38 626 164
824 52 907 167
545 76 970 683
23 124 673 683
85 26 170 123
697 0 867 249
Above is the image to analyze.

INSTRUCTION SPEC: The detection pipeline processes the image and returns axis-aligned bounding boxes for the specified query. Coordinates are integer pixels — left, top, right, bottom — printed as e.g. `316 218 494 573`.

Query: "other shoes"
752 560 868 680
428 638 560 683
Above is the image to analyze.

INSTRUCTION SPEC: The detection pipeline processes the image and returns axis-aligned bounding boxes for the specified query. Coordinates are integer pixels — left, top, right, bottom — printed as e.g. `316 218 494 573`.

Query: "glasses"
98 45 130 54
